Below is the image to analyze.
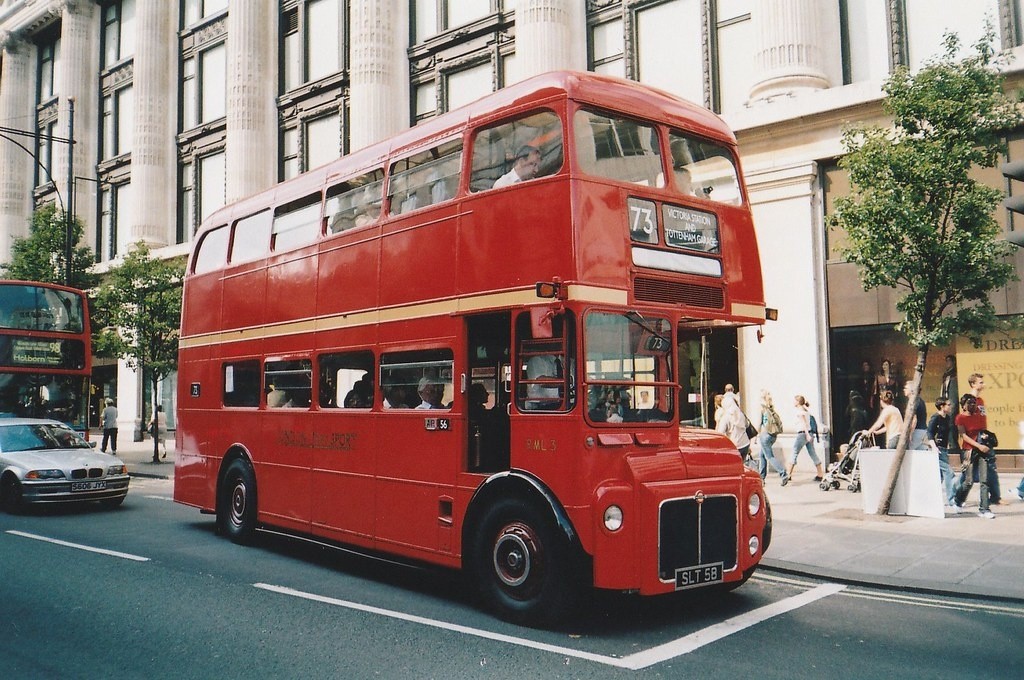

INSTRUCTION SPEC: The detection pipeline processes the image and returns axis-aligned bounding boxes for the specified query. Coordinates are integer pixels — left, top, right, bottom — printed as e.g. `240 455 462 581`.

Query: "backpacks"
766 407 783 435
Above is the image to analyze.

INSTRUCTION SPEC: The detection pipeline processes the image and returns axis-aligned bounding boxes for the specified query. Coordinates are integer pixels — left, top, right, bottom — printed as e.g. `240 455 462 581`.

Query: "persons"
638 391 653 409
492 145 541 189
526 351 604 411
940 355 961 452
829 444 849 474
714 384 750 463
100 399 118 454
922 373 1010 519
754 389 789 487
786 395 823 483
468 383 489 410
859 359 901 449
1016 476 1024 501
354 193 395 227
862 380 928 450
148 405 168 458
344 372 444 409
672 167 710 201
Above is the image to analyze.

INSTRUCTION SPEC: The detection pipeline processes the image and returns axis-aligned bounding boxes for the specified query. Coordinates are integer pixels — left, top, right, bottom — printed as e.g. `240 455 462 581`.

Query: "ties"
554 357 564 403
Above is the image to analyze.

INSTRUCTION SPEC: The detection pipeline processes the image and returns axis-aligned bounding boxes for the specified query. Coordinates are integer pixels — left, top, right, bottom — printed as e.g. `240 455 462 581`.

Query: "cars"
0 416 130 515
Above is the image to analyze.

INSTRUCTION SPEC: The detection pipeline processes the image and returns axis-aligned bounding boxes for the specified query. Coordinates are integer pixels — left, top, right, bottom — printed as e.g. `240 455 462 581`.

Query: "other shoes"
1018 489 1024 500
977 507 996 519
993 497 1011 505
814 476 823 483
782 475 789 486
953 499 964 514
162 453 166 458
112 449 116 454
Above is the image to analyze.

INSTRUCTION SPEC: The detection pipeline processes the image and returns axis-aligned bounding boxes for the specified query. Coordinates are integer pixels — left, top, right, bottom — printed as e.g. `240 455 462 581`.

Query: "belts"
798 431 810 433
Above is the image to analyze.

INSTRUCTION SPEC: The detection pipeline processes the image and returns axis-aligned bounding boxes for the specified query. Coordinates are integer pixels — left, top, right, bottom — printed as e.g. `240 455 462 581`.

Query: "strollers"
819 430 876 494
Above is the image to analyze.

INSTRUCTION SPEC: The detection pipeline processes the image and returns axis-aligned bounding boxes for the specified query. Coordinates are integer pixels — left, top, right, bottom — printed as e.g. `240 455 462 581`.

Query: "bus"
0 280 92 441
173 69 774 631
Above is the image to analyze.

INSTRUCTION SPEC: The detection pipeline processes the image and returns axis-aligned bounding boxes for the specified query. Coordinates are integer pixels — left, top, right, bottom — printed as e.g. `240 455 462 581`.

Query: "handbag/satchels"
147 424 155 433
810 414 820 443
746 422 758 439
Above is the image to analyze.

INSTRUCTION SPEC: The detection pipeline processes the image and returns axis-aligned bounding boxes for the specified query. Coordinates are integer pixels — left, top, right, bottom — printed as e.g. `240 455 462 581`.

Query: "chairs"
327 153 714 235
